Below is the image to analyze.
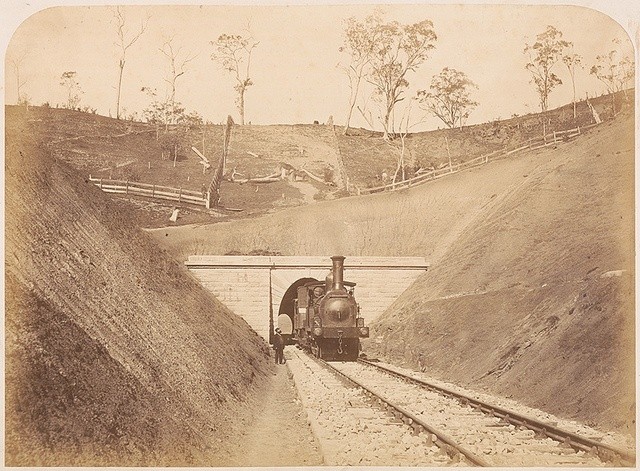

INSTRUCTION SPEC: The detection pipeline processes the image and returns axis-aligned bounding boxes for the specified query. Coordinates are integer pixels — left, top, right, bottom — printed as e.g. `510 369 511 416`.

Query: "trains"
295 255 369 362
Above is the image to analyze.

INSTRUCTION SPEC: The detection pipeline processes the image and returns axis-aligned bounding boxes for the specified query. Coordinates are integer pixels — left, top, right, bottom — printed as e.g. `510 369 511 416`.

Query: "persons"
272 328 287 365
